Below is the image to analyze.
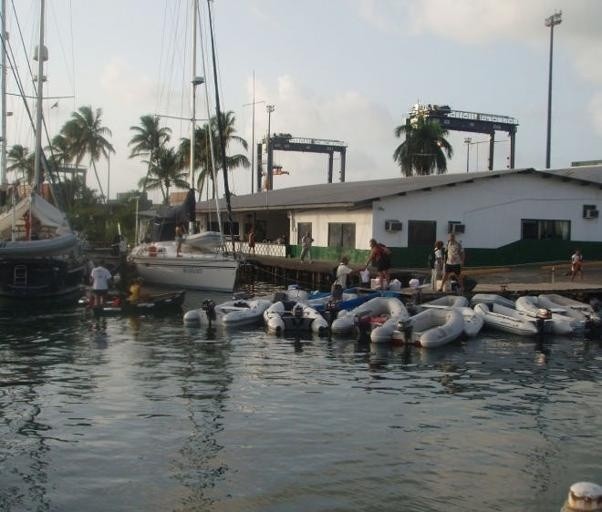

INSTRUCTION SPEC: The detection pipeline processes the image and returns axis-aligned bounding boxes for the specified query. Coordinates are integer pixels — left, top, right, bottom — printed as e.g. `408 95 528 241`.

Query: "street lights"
463 137 473 173
263 105 277 192
544 10 564 170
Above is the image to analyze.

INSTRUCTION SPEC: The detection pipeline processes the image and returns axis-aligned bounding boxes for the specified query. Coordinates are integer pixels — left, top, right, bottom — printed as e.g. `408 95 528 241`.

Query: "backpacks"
428 248 441 267
376 243 392 262
327 264 344 285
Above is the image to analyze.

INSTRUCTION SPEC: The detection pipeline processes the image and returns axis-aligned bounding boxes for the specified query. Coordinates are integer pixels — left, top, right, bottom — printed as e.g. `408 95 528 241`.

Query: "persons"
300 232 315 264
438 233 465 293
175 221 186 257
248 232 257 256
331 257 365 296
91 261 112 304
430 241 443 290
365 239 392 291
567 248 582 281
570 256 583 282
